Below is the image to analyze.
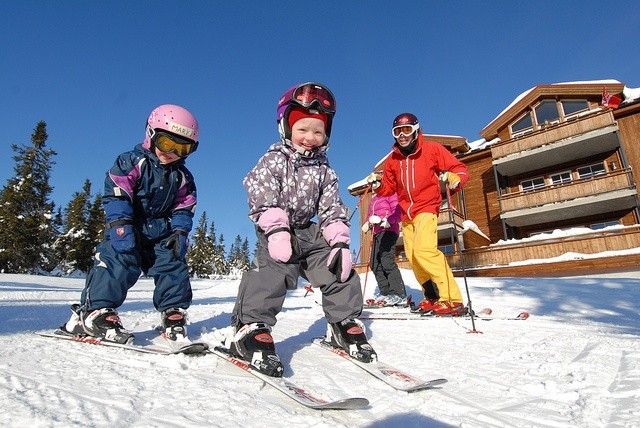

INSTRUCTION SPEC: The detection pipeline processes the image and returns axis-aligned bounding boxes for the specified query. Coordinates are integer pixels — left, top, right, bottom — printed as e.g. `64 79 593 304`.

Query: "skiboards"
206 336 447 409
360 309 529 321
33 325 209 355
362 299 418 307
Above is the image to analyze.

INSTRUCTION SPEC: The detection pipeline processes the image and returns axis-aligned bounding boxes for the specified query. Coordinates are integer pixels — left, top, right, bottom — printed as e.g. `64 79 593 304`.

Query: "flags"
602 85 622 109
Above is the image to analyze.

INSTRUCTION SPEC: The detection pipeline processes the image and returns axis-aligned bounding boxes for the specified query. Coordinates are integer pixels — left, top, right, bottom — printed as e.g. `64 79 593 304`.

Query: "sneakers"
366 293 389 307
161 307 188 344
60 304 135 345
417 298 439 312
431 301 466 317
222 319 284 378
323 317 378 364
377 294 408 307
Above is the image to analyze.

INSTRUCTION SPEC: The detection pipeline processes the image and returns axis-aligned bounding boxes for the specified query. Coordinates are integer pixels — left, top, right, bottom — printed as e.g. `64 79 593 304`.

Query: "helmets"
142 103 200 155
276 84 337 146
392 113 421 149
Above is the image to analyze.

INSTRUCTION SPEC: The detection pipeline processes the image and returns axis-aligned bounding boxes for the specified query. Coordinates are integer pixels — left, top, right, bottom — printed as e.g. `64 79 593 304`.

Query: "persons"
62 104 198 344
223 81 379 379
367 112 471 316
361 173 408 309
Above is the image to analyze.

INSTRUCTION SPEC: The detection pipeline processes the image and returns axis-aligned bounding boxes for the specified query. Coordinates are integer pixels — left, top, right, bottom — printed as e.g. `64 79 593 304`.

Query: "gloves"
258 208 294 264
380 218 392 229
367 172 385 191
439 171 462 191
164 211 195 260
322 221 353 285
362 221 373 235
106 200 138 254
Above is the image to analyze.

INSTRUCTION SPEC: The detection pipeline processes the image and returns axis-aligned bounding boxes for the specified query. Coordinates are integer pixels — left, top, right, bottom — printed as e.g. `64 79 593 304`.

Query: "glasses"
278 84 336 114
392 122 420 139
152 131 195 158
